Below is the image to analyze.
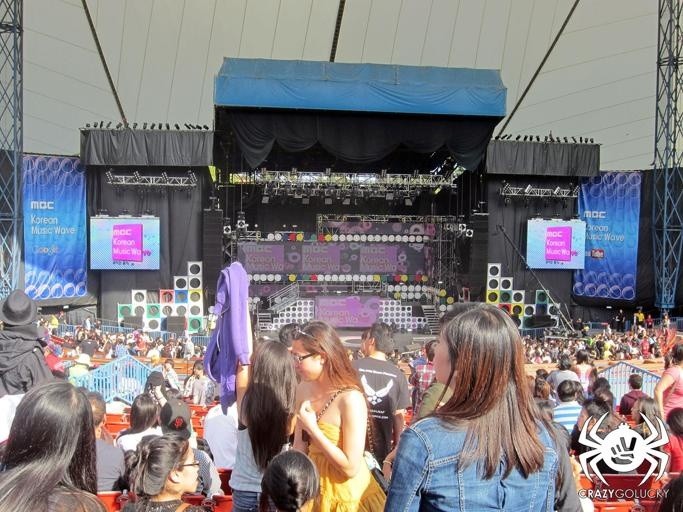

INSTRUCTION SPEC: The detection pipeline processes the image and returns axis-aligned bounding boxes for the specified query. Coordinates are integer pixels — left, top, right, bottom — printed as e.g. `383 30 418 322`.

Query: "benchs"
47 333 682 511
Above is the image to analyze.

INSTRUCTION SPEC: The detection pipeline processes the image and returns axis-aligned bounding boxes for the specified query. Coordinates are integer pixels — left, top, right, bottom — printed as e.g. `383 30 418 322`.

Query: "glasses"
180 459 200 468
290 351 318 362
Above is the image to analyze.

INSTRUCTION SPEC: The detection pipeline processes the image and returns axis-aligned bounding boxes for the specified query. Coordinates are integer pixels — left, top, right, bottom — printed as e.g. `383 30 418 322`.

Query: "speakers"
203 208 223 289
469 216 488 296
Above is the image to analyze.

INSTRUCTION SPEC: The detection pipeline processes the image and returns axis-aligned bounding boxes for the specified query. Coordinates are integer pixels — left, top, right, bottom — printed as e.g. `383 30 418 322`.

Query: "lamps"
500 182 581 210
106 168 197 201
259 167 421 207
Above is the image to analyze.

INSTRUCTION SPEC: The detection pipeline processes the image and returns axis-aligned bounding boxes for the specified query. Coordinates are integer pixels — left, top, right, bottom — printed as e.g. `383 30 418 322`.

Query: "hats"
0 289 37 326
159 400 192 441
146 371 164 386
